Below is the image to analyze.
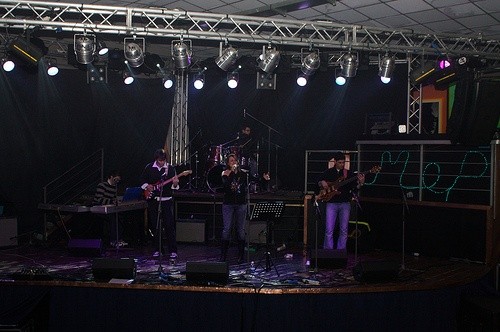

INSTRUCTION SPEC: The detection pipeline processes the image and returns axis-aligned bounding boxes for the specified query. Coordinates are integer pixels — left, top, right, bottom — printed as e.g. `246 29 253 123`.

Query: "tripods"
252 200 285 276
389 184 426 277
179 145 209 192
145 175 175 275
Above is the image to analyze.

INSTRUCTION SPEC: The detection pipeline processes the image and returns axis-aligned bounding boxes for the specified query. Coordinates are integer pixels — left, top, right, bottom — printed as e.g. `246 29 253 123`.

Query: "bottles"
248 261 256 280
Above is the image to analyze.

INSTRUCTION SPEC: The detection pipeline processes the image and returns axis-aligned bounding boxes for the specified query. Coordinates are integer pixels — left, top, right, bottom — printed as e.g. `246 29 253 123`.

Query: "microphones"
244 108 246 118
200 128 202 136
10 237 16 240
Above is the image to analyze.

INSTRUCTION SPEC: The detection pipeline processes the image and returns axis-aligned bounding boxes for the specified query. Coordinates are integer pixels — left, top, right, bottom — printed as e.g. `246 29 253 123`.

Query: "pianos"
31 202 91 251
90 198 163 255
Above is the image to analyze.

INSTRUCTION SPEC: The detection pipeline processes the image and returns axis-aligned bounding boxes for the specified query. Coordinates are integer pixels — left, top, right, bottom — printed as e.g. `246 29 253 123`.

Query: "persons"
315 152 364 249
220 154 270 262
93 171 123 241
141 149 180 255
233 125 255 146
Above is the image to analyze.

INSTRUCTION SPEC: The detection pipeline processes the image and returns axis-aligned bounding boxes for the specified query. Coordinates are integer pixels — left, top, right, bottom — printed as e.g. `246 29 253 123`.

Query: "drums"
205 145 224 163
203 162 225 193
222 146 245 163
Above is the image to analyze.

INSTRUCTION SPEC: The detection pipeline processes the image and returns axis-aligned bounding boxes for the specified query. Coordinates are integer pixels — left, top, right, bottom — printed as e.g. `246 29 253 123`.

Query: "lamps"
411 50 471 89
0 24 395 89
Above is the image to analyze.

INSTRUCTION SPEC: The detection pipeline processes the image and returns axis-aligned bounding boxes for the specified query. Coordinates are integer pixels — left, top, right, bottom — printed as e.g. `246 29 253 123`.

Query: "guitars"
316 166 382 202
144 170 192 200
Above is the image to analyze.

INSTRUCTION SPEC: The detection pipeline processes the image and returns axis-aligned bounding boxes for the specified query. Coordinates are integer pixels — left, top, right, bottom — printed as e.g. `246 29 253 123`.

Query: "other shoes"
170 252 177 260
153 252 159 257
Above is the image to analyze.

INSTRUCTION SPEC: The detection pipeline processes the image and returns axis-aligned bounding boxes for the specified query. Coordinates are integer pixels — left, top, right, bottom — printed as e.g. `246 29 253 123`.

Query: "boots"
235 240 245 263
220 240 228 262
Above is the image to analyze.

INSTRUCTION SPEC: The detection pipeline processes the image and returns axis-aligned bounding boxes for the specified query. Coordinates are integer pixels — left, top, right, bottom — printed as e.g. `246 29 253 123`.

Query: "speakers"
92 258 135 281
0 217 18 247
185 260 229 286
310 248 346 268
